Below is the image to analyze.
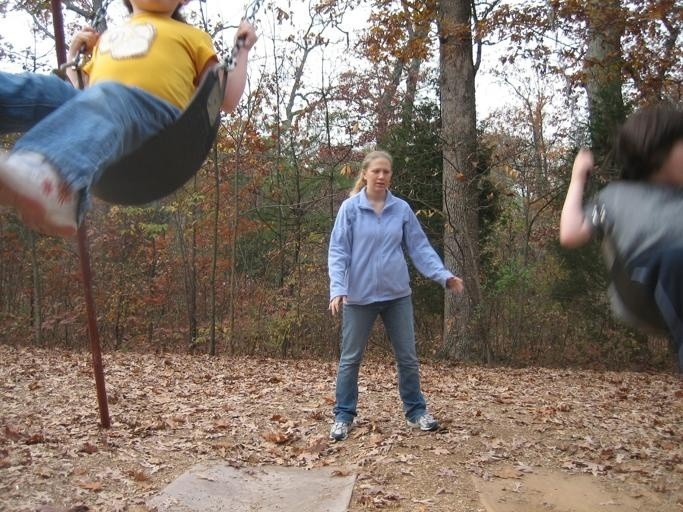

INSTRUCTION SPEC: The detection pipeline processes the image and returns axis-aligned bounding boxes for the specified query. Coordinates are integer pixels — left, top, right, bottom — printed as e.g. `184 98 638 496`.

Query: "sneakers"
1 151 77 237
329 422 352 440
407 413 438 430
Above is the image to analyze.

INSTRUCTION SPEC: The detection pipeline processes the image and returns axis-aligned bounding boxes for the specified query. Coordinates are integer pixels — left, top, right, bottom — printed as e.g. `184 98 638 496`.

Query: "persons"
0 0 259 241
557 103 682 378
323 149 464 442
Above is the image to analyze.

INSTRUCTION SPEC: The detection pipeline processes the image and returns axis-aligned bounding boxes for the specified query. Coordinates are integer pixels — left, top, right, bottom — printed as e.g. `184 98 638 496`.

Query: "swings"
49 0 265 203
584 144 671 337
